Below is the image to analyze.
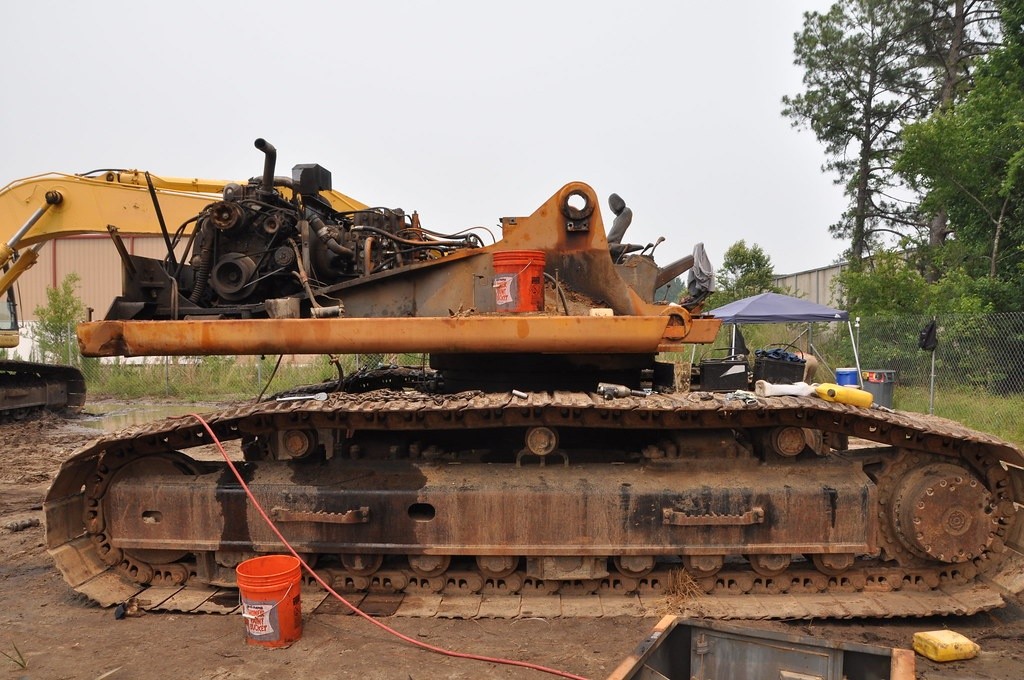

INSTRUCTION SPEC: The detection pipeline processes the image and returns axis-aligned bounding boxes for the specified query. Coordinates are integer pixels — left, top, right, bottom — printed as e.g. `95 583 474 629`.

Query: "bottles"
754 379 814 398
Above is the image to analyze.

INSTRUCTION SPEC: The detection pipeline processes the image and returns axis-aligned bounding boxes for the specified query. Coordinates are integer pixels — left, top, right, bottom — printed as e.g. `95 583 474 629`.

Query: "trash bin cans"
861 370 896 409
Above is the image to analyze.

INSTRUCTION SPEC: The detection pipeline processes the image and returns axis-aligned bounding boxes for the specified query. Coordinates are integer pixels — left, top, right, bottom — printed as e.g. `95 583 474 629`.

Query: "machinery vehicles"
39 135 1024 624
0 161 372 442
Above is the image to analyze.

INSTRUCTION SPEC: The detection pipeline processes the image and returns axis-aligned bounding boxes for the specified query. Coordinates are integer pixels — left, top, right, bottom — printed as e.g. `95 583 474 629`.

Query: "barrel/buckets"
236 554 303 647
492 250 546 312
912 630 979 662
754 342 805 391
835 368 857 386
698 346 748 391
816 382 873 407
861 369 896 409
